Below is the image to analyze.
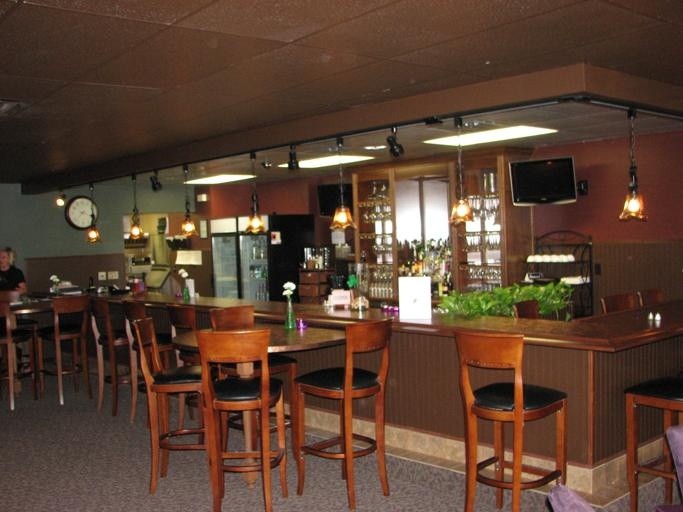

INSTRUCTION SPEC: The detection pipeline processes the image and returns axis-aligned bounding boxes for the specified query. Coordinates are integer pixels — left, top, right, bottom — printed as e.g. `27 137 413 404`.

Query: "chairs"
600 293 635 314
624 374 683 512
637 288 666 307
454 329 568 511
513 300 539 319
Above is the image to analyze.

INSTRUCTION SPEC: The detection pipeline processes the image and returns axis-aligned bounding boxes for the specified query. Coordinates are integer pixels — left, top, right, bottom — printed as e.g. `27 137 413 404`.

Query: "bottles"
397 239 454 298
250 237 269 301
369 282 392 299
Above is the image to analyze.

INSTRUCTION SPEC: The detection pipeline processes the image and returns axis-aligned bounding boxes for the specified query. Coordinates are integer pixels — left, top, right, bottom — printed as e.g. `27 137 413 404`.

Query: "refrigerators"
210 214 270 303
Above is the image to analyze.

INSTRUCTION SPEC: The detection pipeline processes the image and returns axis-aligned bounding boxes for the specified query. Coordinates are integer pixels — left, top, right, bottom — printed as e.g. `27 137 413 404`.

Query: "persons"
0 247 27 296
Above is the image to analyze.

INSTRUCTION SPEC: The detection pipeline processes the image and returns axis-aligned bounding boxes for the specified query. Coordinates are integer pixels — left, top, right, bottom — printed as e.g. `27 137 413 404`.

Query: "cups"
175 287 183 297
296 318 308 330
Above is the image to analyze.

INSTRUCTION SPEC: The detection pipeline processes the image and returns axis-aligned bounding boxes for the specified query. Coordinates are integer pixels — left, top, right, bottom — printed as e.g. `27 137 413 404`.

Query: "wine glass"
462 194 502 281
361 180 391 254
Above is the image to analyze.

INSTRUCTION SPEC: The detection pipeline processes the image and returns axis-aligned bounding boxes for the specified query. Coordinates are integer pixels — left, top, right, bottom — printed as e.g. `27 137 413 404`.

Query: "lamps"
386 126 405 158
448 117 473 224
149 170 162 192
244 138 358 235
56 190 66 207
129 174 144 240
87 184 101 243
618 107 648 222
181 166 199 237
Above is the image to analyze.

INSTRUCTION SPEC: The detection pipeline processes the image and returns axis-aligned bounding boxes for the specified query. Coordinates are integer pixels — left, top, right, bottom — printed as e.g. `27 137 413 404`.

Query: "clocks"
64 196 99 231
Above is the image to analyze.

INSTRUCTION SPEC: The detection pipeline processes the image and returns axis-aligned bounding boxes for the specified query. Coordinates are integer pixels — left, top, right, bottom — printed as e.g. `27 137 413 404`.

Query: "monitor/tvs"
509 156 577 207
317 183 354 219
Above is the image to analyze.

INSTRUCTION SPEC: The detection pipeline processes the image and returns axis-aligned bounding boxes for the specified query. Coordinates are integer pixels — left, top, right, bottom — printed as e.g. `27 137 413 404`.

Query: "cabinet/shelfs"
526 230 594 318
357 199 394 283
458 192 503 282
298 271 337 304
